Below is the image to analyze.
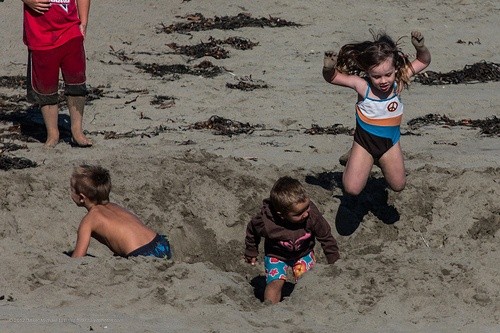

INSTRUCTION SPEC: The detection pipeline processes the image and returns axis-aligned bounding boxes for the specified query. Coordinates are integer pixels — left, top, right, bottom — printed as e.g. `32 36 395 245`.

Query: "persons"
245 176 342 306
70 165 173 260
323 29 431 194
20 0 93 147
